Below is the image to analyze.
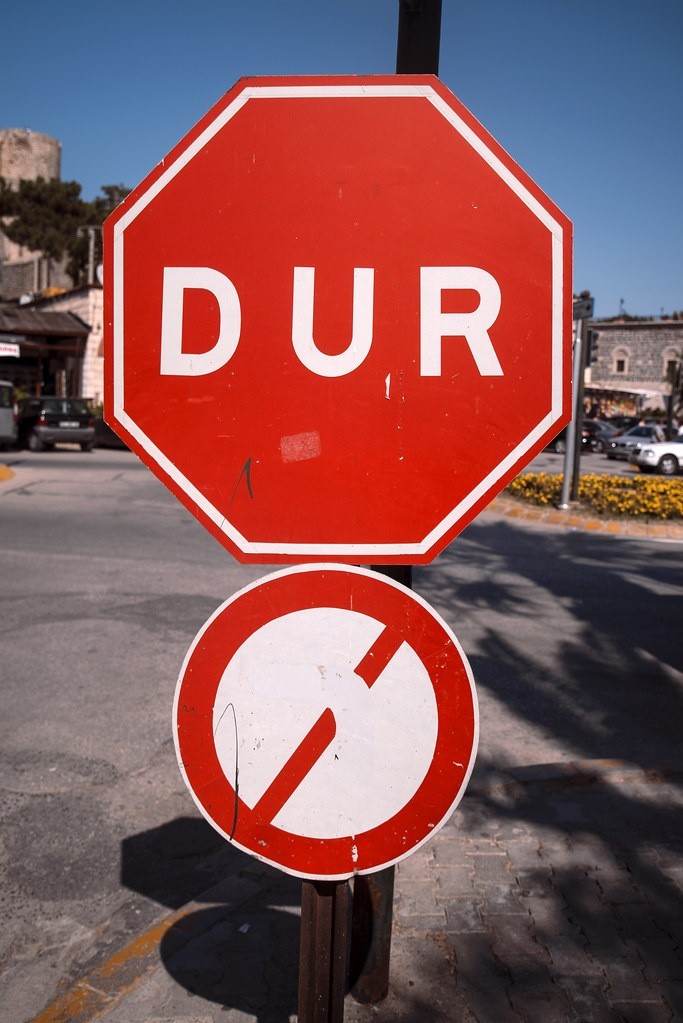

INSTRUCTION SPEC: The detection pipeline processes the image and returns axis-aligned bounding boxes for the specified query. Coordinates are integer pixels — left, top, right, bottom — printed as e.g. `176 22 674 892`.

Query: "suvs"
16 396 96 451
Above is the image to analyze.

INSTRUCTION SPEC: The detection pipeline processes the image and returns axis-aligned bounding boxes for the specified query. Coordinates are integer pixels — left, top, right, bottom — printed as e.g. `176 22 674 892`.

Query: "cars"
631 432 683 474
583 417 626 453
606 423 677 461
548 424 593 453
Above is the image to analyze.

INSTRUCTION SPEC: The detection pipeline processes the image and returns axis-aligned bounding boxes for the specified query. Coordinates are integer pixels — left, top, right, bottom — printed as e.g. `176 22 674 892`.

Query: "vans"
0 380 19 444
